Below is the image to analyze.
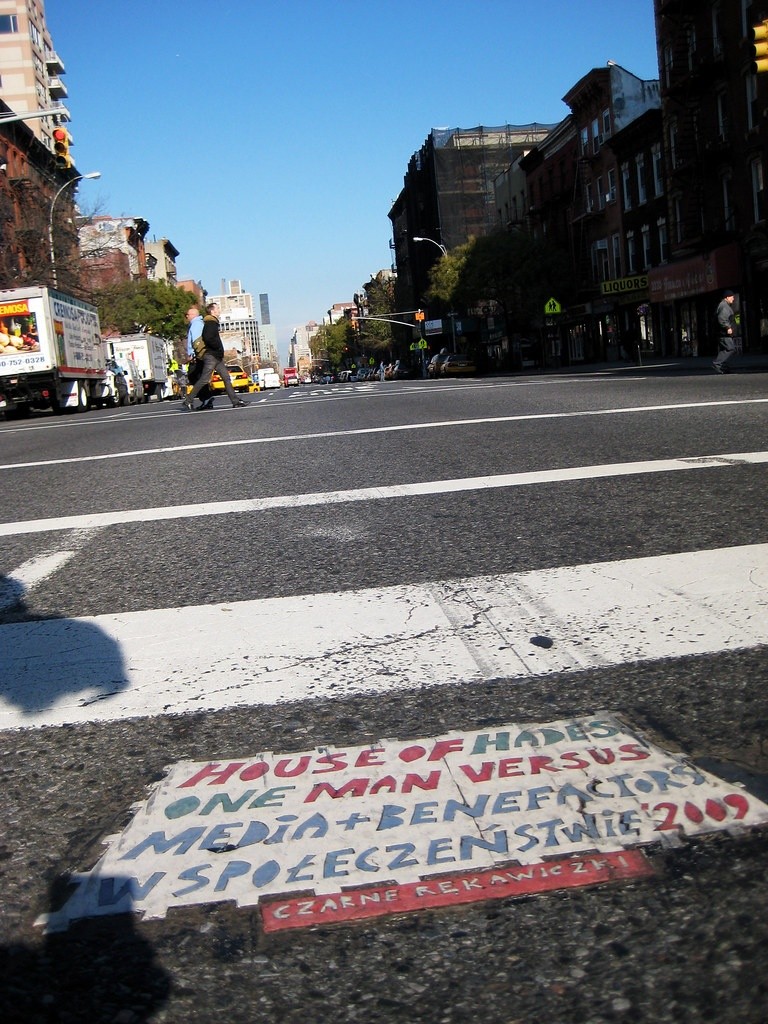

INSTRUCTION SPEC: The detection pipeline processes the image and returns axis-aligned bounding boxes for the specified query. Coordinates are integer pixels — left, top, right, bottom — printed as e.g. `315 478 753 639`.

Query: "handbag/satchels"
193 336 207 361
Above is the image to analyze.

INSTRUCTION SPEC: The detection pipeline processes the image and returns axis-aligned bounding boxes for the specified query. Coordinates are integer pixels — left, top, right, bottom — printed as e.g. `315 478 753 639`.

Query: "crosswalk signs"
370 358 374 364
545 298 561 313
418 339 427 349
410 343 418 350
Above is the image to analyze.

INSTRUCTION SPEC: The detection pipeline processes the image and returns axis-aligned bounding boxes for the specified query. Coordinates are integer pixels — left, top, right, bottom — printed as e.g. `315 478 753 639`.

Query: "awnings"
648 241 744 304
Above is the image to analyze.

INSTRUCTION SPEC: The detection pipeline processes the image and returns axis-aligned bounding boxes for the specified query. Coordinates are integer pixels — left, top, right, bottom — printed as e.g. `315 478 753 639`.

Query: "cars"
209 365 249 396
114 358 145 404
426 347 451 379
287 376 299 387
439 353 476 378
299 360 411 384
88 359 130 409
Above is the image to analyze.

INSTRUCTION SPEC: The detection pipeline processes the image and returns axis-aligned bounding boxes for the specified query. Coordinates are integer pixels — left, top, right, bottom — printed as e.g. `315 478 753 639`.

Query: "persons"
711 290 737 375
181 303 251 412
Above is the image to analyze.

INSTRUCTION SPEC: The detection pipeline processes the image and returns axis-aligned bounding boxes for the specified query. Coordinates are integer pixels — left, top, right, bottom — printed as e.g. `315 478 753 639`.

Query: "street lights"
413 237 456 355
49 172 102 290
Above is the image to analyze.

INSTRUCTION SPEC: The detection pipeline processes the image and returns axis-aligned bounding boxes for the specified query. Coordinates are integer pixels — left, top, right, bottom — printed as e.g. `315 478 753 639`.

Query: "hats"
724 290 734 298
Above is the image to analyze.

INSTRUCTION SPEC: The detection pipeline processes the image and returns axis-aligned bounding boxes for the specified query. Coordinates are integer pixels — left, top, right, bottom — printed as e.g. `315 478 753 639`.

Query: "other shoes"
196 398 215 410
712 365 733 375
182 401 195 411
233 399 250 408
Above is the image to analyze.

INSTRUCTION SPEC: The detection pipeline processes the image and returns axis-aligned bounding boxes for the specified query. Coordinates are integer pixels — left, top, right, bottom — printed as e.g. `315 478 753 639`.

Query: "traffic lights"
53 127 71 169
352 321 355 330
748 19 768 74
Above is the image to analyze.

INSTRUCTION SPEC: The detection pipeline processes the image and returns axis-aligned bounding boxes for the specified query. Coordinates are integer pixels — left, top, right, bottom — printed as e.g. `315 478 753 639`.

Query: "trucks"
102 333 172 403
258 368 297 391
0 285 114 421
